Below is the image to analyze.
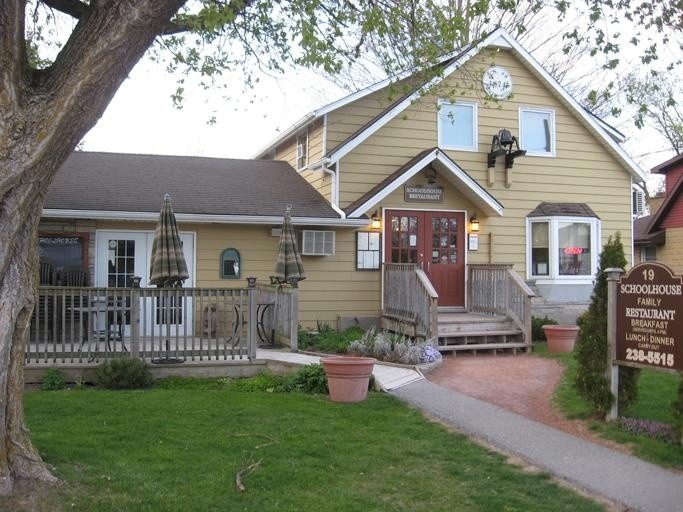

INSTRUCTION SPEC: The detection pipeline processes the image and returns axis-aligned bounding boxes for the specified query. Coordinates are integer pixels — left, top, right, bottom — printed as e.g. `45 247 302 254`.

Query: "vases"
541 324 580 352
321 357 378 403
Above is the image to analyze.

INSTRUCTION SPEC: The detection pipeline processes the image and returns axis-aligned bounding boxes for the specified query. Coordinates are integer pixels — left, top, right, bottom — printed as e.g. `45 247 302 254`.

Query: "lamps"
470 213 480 231
426 163 437 184
370 210 381 229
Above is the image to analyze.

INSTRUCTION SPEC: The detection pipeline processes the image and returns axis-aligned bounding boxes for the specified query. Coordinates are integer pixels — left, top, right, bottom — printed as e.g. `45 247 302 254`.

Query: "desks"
224 302 274 348
67 307 131 360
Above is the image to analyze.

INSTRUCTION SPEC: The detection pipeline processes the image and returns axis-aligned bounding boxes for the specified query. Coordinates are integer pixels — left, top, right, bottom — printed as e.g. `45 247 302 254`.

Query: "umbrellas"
268 204 306 344
147 193 189 361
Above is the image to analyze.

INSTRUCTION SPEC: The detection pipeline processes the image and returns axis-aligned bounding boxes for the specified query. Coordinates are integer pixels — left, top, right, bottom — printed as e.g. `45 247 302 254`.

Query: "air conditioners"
297 230 336 256
633 191 645 222
297 144 306 160
